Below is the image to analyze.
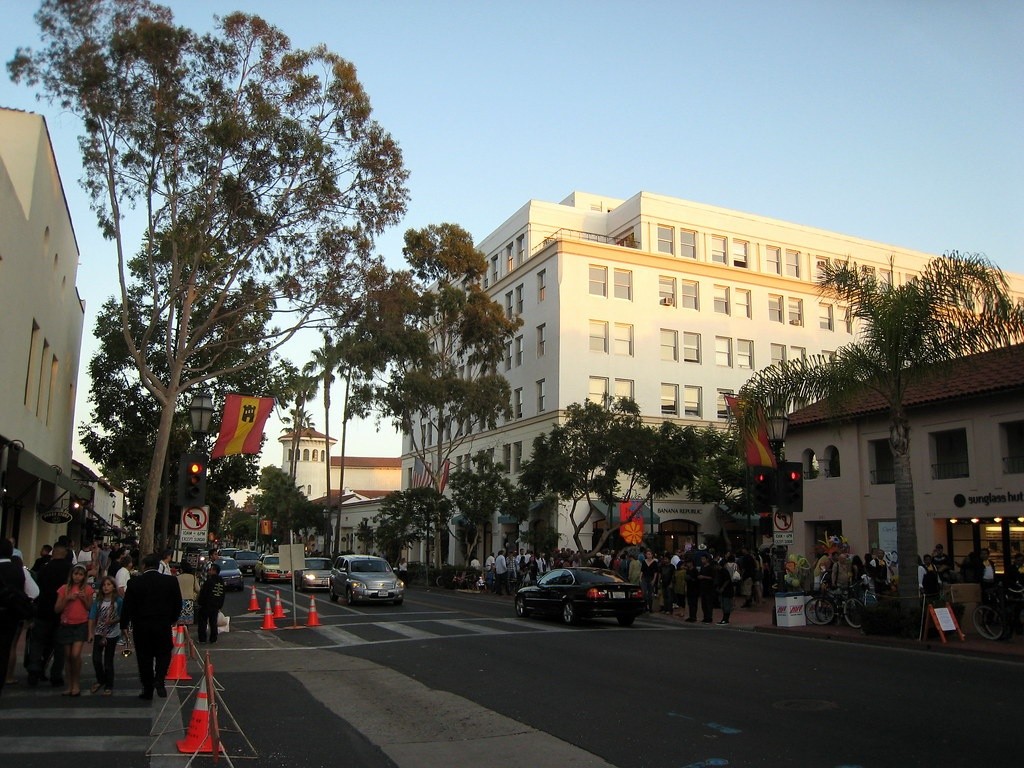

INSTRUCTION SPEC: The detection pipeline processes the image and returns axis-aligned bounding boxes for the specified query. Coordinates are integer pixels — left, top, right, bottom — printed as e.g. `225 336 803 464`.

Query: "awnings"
718 503 759 526
590 500 660 524
497 500 543 525
451 508 492 524
0 435 91 514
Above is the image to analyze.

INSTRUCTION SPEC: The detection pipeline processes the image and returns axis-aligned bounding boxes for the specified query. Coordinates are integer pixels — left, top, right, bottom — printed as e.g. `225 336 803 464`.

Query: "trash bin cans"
774 591 807 628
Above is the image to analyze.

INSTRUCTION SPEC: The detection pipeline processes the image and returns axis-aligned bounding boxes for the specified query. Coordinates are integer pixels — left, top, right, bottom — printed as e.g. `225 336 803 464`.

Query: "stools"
775 590 807 627
804 591 823 624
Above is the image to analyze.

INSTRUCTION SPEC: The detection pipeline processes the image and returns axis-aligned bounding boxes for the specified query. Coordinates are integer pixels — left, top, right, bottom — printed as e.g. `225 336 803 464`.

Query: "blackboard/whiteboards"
925 604 959 632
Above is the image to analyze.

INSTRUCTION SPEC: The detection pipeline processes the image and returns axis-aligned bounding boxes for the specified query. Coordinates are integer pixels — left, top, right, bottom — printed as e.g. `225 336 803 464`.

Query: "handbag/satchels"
524 573 530 582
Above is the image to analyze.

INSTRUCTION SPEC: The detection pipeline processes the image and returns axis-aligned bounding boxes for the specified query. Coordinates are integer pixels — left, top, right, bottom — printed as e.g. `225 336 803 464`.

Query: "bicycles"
973 579 1024 641
805 571 886 626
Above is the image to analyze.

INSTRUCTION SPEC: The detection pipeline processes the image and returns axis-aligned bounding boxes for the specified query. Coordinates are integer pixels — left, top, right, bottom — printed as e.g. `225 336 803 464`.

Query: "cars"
197 548 239 559
295 557 333 590
255 554 292 585
515 567 644 625
232 550 260 575
329 555 406 608
214 557 245 591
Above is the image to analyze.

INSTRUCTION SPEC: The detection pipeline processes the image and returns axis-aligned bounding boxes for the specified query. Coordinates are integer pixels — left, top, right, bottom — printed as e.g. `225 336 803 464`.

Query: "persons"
0 535 226 700
470 543 776 626
827 544 1024 639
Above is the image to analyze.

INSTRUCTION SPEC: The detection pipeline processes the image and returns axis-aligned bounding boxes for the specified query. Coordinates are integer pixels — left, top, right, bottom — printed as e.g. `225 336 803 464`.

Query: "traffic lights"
188 463 206 503
755 470 771 512
789 471 803 505
760 517 773 536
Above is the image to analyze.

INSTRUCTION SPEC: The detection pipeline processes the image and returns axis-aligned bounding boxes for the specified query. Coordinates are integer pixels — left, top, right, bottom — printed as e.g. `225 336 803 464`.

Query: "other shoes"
103 687 112 696
140 694 153 700
92 682 104 694
61 691 71 696
700 620 711 624
716 619 730 625
152 681 167 697
70 688 81 696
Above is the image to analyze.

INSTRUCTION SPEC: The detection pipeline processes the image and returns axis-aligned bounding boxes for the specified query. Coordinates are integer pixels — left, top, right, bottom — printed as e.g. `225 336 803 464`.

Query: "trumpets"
121 633 133 658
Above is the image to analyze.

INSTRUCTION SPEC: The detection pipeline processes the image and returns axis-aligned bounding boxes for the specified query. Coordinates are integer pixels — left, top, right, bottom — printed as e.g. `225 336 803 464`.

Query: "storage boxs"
945 583 982 603
960 602 979 634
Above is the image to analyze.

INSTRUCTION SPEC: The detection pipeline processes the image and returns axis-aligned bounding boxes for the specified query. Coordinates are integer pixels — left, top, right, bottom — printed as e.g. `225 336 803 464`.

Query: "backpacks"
921 564 937 598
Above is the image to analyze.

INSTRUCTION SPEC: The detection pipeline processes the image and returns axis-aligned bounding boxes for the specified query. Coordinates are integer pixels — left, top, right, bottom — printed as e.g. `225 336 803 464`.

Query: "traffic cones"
248 585 261 611
260 597 278 630
163 626 193 680
304 596 322 627
174 677 234 755
273 590 287 619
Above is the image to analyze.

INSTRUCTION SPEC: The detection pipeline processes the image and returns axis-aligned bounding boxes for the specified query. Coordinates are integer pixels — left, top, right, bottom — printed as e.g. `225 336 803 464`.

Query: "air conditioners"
818 260 826 267
792 320 800 325
663 297 673 305
864 266 874 275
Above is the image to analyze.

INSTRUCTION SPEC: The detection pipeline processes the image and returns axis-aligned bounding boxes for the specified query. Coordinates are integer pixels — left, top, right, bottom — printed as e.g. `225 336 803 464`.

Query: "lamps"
950 518 958 524
971 518 980 524
1017 517 1024 522
993 518 1003 522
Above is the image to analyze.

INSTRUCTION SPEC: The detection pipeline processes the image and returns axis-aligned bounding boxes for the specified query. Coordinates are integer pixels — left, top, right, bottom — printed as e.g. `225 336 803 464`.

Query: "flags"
212 395 274 458
411 453 450 494
725 396 779 469
